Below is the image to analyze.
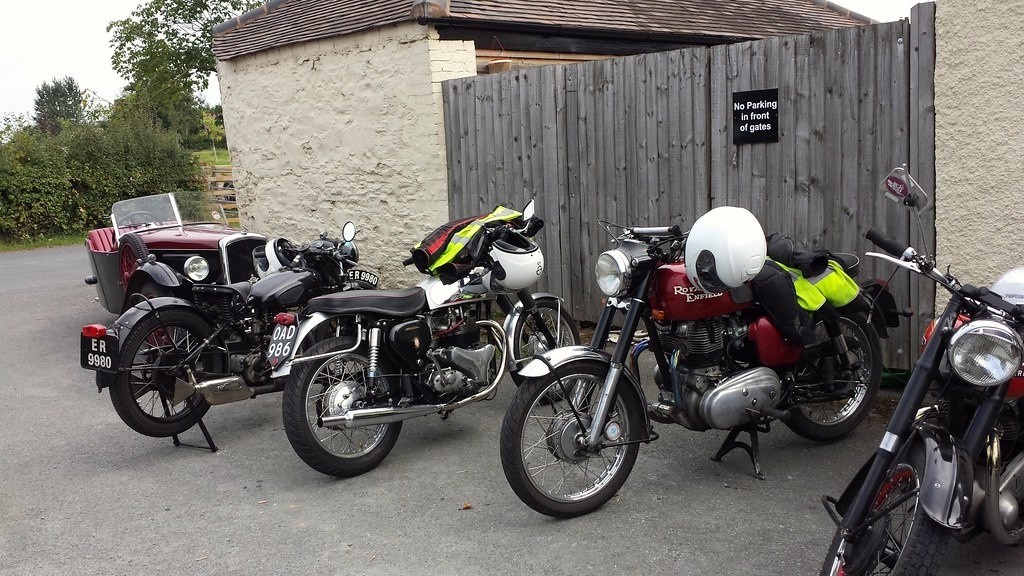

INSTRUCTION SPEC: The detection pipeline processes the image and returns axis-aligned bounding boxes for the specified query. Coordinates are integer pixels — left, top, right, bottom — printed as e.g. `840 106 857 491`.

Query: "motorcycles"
498 206 915 520
79 219 385 454
265 199 584 478
818 162 1024 576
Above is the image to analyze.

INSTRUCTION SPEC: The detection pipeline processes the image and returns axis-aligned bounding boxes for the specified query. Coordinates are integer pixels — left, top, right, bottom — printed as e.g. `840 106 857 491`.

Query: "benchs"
86 220 190 252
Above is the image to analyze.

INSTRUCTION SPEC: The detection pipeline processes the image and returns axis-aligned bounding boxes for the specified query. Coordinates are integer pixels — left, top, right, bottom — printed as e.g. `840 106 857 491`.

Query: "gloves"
769 232 829 278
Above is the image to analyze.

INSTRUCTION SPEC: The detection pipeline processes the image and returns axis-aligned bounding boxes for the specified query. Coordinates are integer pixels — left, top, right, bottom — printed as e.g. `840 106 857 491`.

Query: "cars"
84 190 269 321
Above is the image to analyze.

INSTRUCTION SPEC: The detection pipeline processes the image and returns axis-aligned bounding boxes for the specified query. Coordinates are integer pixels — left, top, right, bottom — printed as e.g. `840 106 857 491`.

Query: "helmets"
684 205 767 294
486 228 545 290
252 237 290 278
991 268 1023 307
